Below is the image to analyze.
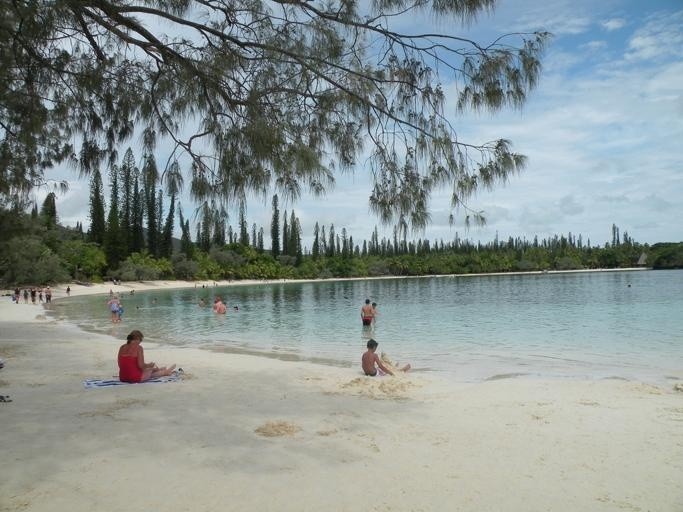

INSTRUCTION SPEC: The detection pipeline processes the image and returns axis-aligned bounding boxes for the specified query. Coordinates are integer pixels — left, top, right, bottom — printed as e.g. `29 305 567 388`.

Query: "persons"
360 338 411 376
360 299 376 326
106 287 136 323
65 286 70 296
371 301 379 316
197 295 239 315
11 285 52 305
117 329 177 383
